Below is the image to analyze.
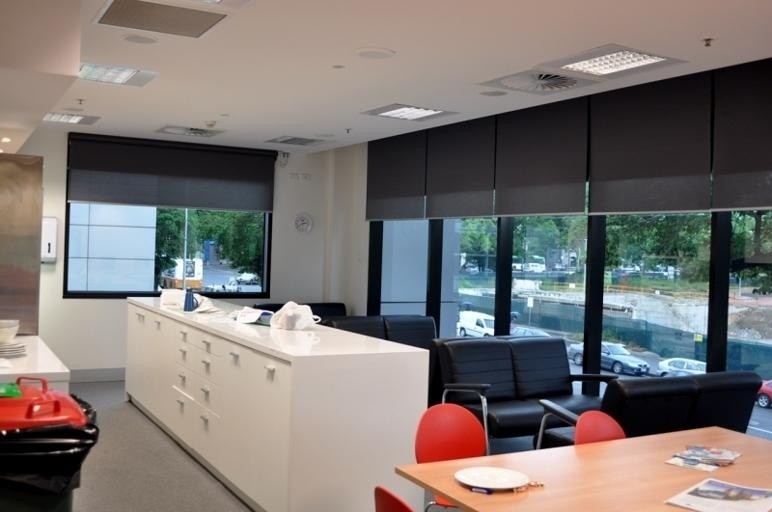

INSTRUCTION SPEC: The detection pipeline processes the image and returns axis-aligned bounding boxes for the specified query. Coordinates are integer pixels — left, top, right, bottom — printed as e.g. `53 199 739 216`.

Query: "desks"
0 335 70 396
395 425 772 512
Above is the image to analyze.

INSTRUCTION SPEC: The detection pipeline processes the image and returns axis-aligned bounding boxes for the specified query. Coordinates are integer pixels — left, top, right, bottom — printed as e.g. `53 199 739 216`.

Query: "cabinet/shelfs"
125 296 431 512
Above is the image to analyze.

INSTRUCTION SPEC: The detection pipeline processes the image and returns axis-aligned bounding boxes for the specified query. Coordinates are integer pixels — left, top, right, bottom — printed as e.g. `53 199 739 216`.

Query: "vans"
459 310 495 337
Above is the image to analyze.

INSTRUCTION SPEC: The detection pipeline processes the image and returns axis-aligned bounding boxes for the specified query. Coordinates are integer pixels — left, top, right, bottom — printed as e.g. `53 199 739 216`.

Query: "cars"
658 358 707 377
223 279 263 294
757 380 772 408
568 341 650 374
510 327 548 336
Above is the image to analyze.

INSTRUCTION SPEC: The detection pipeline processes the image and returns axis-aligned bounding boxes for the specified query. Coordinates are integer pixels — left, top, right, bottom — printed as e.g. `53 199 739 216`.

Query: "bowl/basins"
0 319 20 344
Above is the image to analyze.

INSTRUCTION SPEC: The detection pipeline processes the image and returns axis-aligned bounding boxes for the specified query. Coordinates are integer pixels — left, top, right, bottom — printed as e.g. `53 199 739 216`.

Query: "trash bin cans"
0 377 100 512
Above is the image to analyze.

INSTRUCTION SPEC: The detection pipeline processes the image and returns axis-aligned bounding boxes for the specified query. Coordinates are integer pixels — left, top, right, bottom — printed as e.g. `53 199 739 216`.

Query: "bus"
457 252 546 273
159 257 203 289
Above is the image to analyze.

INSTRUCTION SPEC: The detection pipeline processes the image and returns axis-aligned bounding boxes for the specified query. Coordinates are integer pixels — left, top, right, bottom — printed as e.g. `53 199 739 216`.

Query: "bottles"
184 287 193 311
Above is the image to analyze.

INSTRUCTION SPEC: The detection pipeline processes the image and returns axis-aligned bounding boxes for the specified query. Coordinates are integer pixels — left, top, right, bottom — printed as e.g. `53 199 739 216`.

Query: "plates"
0 344 26 358
455 466 529 491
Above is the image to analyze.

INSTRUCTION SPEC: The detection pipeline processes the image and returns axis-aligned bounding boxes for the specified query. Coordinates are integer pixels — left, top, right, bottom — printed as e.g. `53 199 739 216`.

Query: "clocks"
294 214 312 235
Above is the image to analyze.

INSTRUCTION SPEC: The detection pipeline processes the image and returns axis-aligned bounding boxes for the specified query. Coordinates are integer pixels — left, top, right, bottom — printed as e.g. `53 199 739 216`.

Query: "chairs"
375 486 414 512
415 403 486 512
